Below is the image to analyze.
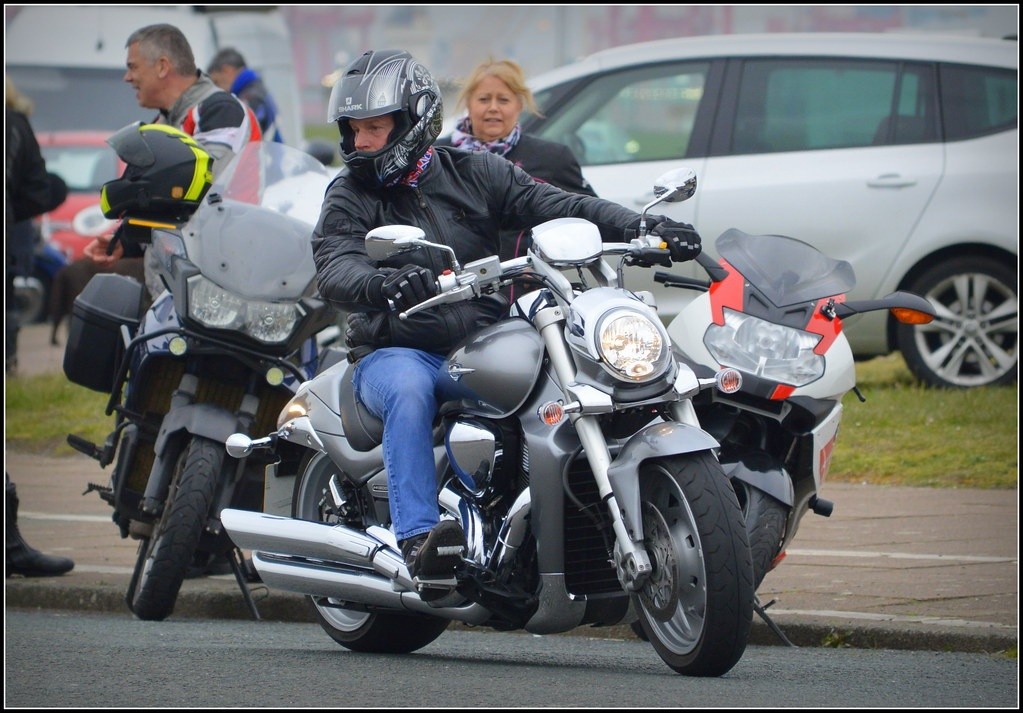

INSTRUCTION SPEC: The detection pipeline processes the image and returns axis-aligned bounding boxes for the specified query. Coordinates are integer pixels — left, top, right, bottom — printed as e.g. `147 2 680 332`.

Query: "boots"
6 482 74 577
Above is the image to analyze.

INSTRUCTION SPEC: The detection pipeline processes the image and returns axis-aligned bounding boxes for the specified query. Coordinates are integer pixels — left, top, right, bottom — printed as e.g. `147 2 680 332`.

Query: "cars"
31 129 126 267
432 29 1018 392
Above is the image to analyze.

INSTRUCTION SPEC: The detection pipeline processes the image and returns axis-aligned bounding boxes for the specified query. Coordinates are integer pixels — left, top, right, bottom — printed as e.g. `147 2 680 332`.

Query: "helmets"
100 122 213 220
332 49 444 188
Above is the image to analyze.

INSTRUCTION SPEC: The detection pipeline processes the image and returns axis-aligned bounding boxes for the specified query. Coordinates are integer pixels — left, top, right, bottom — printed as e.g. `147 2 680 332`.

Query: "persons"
7 21 610 581
310 48 701 602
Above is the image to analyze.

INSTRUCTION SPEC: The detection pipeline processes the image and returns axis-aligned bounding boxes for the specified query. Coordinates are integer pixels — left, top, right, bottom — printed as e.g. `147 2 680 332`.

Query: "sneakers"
400 519 468 601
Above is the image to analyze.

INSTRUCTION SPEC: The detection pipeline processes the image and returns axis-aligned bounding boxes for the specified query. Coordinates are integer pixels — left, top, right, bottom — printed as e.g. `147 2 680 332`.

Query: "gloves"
651 219 702 264
381 262 439 311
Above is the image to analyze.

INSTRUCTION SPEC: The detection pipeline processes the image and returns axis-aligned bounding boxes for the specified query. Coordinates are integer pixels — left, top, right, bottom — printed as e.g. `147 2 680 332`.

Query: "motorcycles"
61 142 945 677
